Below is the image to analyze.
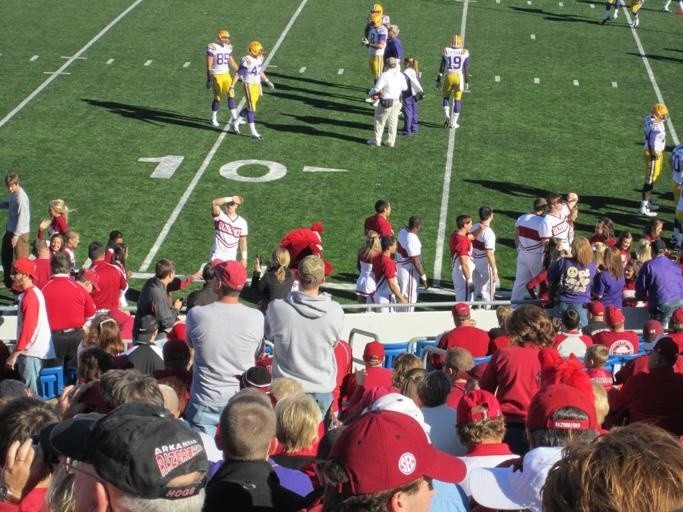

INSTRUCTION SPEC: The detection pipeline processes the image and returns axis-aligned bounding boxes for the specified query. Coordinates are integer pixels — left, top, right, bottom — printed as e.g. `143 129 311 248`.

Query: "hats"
216 260 247 291
11 259 40 280
132 314 159 345
607 306 623 325
541 365 597 404
589 299 607 316
233 368 278 403
364 340 382 362
373 392 431 445
468 447 568 512
468 361 487 381
458 390 503 423
81 266 101 293
51 402 208 499
643 321 663 343
0 380 26 399
645 335 679 362
527 384 600 433
110 231 122 240
387 57 397 68
671 308 683 324
327 411 466 496
453 304 470 317
384 23 399 36
649 240 665 251
297 255 326 283
168 323 186 345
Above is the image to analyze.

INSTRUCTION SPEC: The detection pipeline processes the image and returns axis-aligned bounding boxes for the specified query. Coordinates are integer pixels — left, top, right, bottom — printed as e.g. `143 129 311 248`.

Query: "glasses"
65 456 113 512
49 208 54 212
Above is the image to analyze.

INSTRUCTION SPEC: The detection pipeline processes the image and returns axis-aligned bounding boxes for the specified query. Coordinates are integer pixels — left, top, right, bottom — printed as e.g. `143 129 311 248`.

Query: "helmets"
367 13 381 27
652 102 669 121
249 41 263 57
450 35 463 49
371 3 384 16
216 31 230 44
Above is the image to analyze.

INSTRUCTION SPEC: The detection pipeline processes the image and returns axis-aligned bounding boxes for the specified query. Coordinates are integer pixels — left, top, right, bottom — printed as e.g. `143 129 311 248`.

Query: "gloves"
435 80 442 91
361 39 369 47
206 77 213 89
229 87 235 98
265 79 274 90
464 83 469 91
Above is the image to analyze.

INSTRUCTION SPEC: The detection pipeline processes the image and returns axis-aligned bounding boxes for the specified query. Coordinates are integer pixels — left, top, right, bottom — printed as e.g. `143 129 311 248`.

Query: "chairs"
565 356 617 377
620 348 647 371
415 339 436 357
418 344 492 366
36 368 67 399
382 342 409 371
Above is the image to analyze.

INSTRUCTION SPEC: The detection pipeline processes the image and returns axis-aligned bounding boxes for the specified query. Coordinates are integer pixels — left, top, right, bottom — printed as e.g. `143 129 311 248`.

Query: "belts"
52 328 82 335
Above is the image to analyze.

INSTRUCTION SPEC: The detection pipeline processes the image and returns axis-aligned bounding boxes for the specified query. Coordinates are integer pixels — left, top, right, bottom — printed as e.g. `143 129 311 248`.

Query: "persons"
360 13 388 108
364 200 395 239
383 24 403 70
268 378 302 405
52 230 80 274
592 244 609 269
49 199 69 233
365 237 408 313
264 254 343 424
486 306 514 338
317 411 467 512
537 421 682 512
156 341 194 397
543 192 578 261
435 35 470 130
634 240 683 335
469 206 501 310
580 379 609 423
621 259 650 308
204 389 314 496
104 233 128 269
304 426 349 512
478 306 561 450
601 0 620 24
1 172 31 288
228 41 274 140
580 301 612 335
108 244 130 314
30 238 53 289
607 337 683 440
118 316 170 380
666 308 683 376
660 141 683 229
269 394 334 512
551 308 593 359
441 346 506 442
185 259 264 437
85 315 123 355
392 352 428 407
401 368 427 407
593 247 625 307
547 237 598 328
394 217 427 313
397 58 424 135
628 0 643 28
641 319 664 344
0 369 207 512
636 103 669 217
637 219 670 262
204 397 305 512
565 203 578 245
471 383 610 512
242 366 273 395
341 342 398 421
609 231 632 267
6 257 51 397
416 370 472 457
38 219 62 250
274 224 333 278
429 303 490 368
588 218 615 247
659 0 683 14
206 30 246 127
448 215 475 309
368 57 408 147
210 195 247 270
187 260 217 310
79 241 126 325
171 315 188 340
581 345 613 391
592 305 638 356
359 394 465 512
41 250 96 386
355 231 384 304
133 258 203 346
366 3 390 24
510 198 552 300
452 388 523 512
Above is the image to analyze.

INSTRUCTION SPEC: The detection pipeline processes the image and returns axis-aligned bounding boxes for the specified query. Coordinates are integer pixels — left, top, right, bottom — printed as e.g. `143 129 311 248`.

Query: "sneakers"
635 22 639 28
209 119 220 126
365 140 381 146
613 13 618 19
230 123 241 135
648 203 659 211
603 14 610 21
670 234 679 243
674 243 683 250
638 207 658 217
629 22 635 28
662 8 672 13
371 99 380 107
364 98 375 103
443 116 450 129
232 119 247 125
383 141 394 148
449 123 460 129
0 280 9 287
250 132 263 141
398 130 410 135
